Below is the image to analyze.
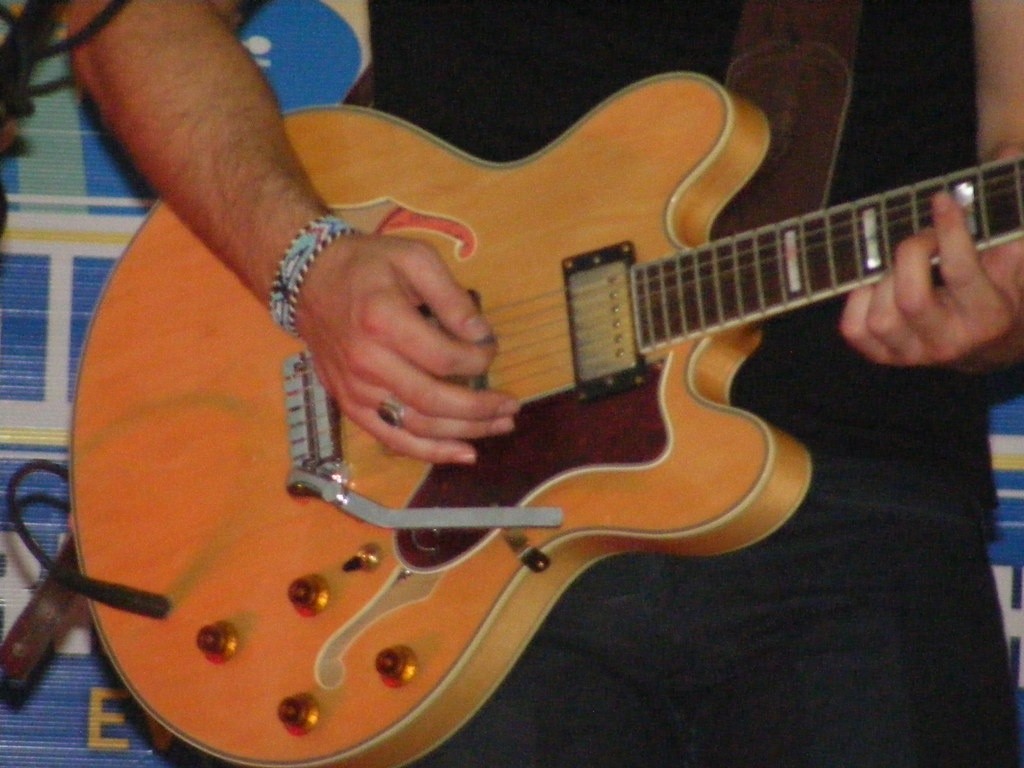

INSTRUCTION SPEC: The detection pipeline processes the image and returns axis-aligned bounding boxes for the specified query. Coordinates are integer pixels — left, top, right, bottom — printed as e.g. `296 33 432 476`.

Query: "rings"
378 393 409 430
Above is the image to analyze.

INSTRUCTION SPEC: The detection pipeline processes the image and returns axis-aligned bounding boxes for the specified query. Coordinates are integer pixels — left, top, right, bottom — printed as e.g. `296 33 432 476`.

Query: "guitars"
69 67 1022 768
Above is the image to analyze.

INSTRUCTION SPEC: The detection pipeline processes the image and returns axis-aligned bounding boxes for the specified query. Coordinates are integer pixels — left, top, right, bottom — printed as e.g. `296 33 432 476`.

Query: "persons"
64 0 1024 767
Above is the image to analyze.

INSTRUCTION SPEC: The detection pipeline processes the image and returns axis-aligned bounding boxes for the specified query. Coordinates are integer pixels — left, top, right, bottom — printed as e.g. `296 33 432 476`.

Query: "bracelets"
269 214 359 337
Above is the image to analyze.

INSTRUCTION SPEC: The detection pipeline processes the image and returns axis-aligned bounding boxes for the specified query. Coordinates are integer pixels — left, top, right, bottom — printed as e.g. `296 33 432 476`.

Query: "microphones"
48 562 171 618
